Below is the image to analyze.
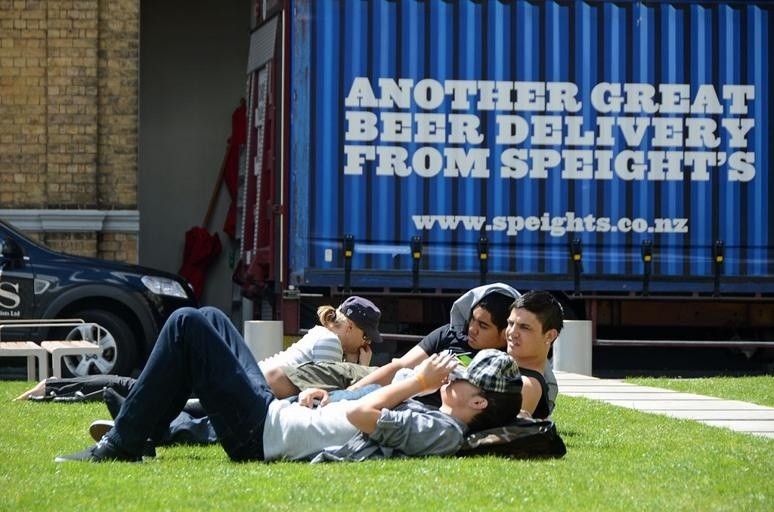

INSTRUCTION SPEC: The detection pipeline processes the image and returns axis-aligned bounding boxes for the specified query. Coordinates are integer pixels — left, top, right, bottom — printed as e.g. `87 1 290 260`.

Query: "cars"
0 217 197 391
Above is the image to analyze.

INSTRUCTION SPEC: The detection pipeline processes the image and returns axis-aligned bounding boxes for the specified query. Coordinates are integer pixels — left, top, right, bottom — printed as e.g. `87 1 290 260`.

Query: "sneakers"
55 420 155 464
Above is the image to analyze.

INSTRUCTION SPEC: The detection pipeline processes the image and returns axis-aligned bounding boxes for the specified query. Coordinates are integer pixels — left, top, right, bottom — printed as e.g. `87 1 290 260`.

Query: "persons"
55 289 563 462
11 294 385 405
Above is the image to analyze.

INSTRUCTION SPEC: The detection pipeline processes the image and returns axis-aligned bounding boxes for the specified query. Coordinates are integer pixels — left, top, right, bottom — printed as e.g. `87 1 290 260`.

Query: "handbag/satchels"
457 420 566 459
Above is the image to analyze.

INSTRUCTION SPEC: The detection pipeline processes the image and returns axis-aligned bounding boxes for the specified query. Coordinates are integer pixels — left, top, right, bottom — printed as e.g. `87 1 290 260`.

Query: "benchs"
0 319 100 382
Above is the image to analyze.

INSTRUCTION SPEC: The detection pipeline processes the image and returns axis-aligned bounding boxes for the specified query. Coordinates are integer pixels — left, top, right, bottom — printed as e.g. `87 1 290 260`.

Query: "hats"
451 348 523 394
340 296 383 343
